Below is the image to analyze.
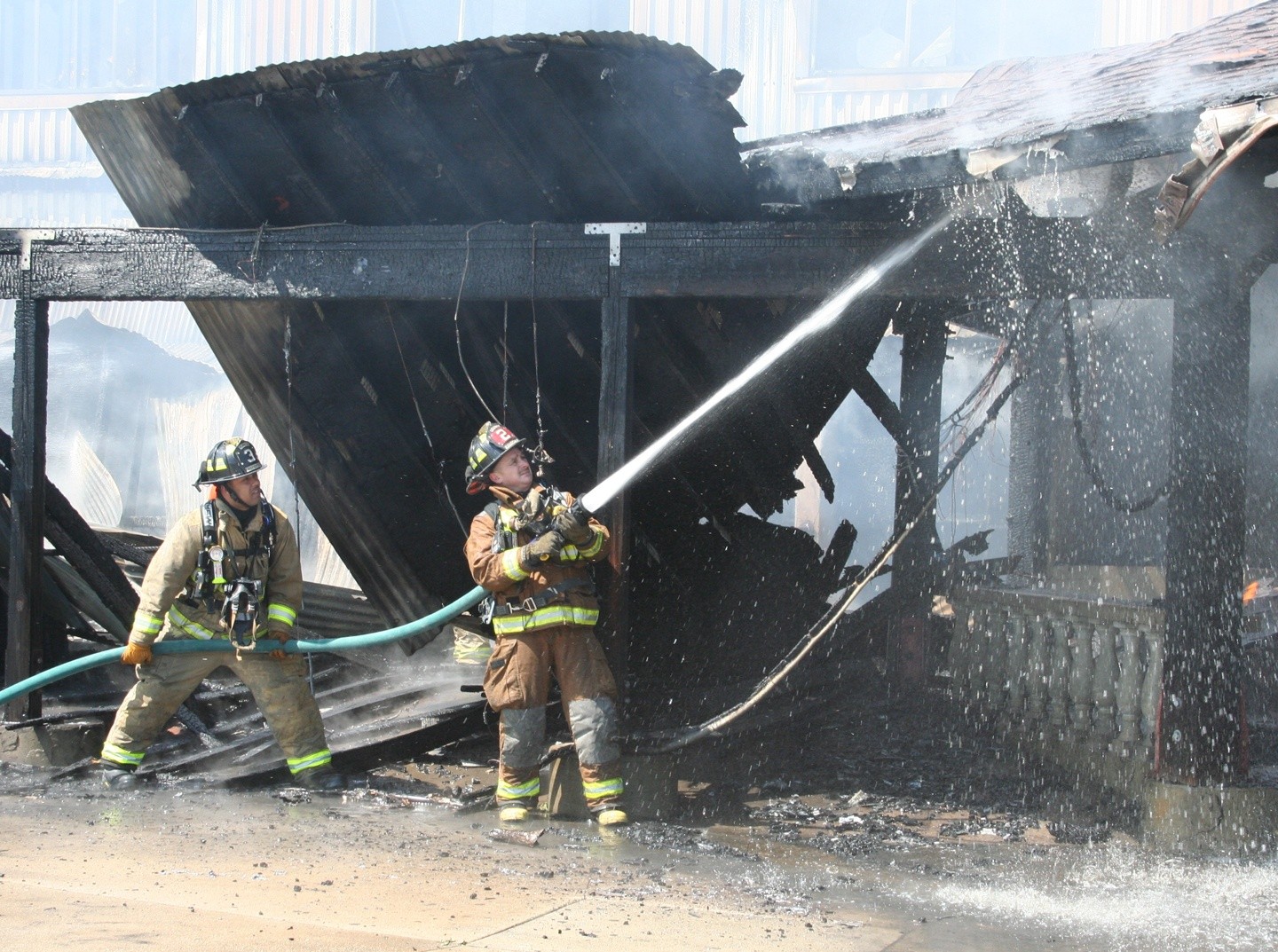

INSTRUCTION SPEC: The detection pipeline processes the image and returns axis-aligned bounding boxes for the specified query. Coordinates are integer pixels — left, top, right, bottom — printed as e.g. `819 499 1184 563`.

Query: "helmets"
189 437 268 487
464 422 526 497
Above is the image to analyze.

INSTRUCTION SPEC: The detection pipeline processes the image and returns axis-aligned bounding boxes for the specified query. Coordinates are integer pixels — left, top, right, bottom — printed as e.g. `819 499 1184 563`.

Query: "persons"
95 439 349 797
459 418 631 825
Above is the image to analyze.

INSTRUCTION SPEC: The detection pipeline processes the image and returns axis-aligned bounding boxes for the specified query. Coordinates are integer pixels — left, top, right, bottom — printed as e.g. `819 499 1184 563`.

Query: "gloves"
120 644 152 666
554 512 593 545
266 634 292 658
526 531 565 566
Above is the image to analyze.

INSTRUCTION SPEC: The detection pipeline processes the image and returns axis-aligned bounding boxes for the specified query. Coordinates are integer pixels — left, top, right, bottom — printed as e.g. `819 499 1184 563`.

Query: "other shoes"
497 805 530 823
295 771 348 790
101 770 142 792
595 810 629 827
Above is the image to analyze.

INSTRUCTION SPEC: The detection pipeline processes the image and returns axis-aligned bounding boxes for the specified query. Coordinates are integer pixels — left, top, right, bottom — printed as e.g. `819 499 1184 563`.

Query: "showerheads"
541 490 596 540
